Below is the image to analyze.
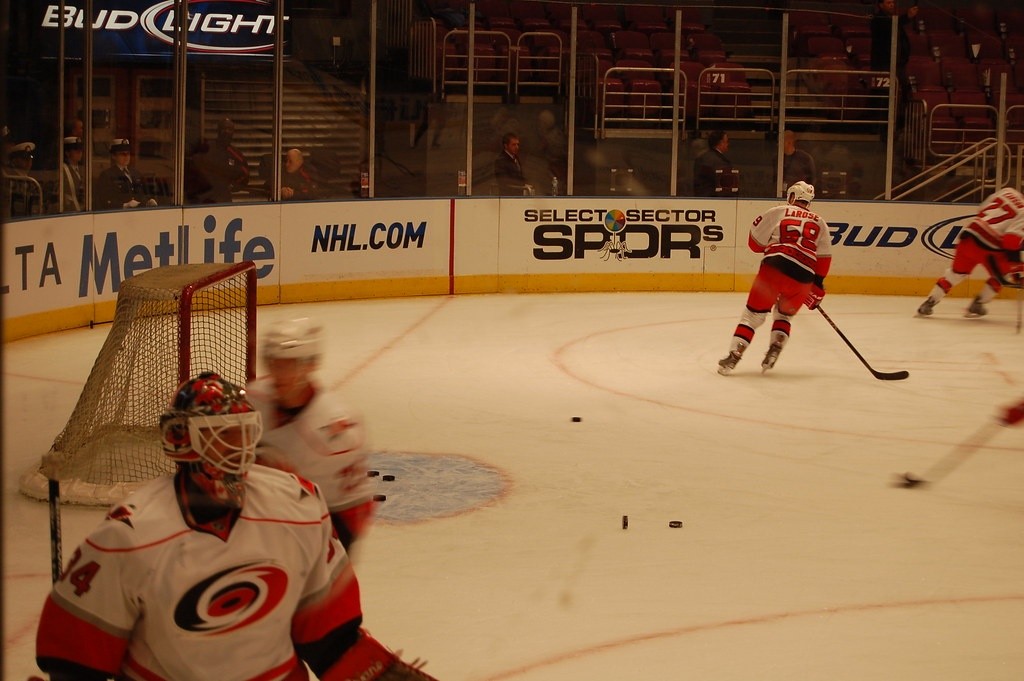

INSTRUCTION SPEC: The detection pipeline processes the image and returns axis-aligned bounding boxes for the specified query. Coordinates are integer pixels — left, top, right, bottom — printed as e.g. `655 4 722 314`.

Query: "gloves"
1010 262 1024 280
123 199 139 209
147 198 158 206
803 283 824 311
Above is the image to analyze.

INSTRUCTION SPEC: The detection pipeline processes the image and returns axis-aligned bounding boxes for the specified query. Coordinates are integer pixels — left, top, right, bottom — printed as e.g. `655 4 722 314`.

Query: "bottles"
360 172 368 197
552 177 559 197
458 170 466 195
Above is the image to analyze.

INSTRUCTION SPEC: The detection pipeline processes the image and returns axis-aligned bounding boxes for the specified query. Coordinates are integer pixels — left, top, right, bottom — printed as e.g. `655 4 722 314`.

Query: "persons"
0 125 42 217
235 315 379 555
211 117 249 193
870 0 917 147
182 137 232 204
693 130 742 198
93 138 157 210
773 129 816 203
265 148 321 202
918 184 1024 319
34 371 439 681
495 132 529 197
52 117 86 212
718 181 832 370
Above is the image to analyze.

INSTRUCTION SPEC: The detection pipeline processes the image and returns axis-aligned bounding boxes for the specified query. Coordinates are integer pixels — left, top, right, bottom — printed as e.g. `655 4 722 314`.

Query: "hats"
7 142 35 161
109 139 131 151
65 137 83 149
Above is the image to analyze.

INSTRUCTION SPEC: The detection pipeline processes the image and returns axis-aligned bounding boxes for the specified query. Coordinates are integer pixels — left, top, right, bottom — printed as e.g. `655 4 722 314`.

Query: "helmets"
159 372 261 509
263 317 321 365
787 181 814 207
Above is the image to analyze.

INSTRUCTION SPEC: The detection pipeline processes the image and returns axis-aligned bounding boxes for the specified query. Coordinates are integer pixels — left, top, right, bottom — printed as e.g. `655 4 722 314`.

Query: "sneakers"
914 296 939 318
719 343 745 376
761 334 785 373
965 295 986 319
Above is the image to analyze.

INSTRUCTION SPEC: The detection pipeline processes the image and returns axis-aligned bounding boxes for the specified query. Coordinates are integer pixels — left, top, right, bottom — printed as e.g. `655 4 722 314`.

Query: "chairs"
0 0 372 199
411 1 1024 158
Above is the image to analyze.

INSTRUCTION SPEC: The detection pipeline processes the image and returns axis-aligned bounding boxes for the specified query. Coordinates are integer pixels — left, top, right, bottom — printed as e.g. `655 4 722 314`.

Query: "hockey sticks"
899 421 1003 488
816 304 910 381
38 452 66 585
987 253 1024 289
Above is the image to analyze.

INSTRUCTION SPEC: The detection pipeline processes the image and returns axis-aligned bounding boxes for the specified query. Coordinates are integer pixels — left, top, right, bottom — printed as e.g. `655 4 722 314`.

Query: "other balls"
573 417 580 422
374 495 386 501
669 521 682 528
367 471 379 477
623 516 628 529
383 475 395 481
